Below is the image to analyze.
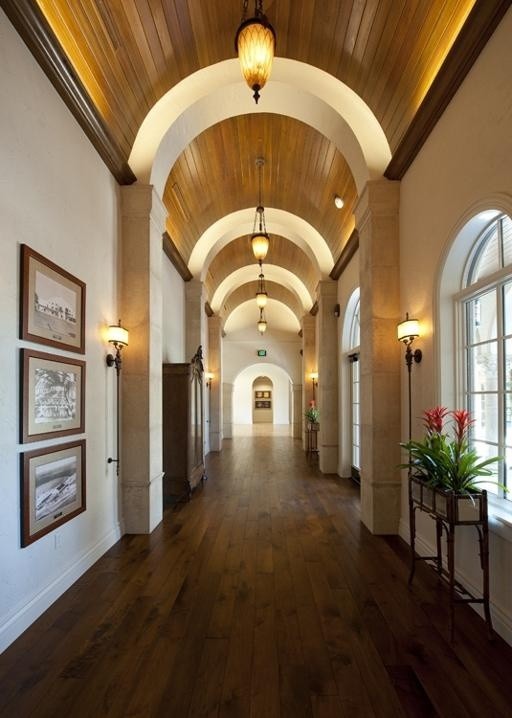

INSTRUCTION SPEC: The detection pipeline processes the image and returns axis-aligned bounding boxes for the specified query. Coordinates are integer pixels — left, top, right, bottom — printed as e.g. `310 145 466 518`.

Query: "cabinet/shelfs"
402 472 499 649
305 421 320 462
160 344 210 504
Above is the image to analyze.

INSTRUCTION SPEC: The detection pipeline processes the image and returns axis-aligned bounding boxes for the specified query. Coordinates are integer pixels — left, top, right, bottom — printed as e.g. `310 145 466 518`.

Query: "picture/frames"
254 390 271 409
15 345 86 446
18 238 90 357
229 0 279 107
16 437 87 551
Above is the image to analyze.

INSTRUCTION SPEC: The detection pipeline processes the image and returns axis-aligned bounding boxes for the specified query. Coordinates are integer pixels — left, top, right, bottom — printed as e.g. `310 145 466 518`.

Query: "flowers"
391 399 512 508
304 400 320 422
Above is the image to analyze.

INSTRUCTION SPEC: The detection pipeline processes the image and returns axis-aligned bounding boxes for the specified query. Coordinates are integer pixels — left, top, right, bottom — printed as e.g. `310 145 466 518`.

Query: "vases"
410 474 482 522
307 422 319 431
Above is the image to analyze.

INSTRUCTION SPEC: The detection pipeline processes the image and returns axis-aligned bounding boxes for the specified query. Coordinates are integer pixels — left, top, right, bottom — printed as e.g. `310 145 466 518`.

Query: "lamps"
392 309 424 542
246 154 277 337
103 315 130 478
308 371 318 402
205 373 215 392
